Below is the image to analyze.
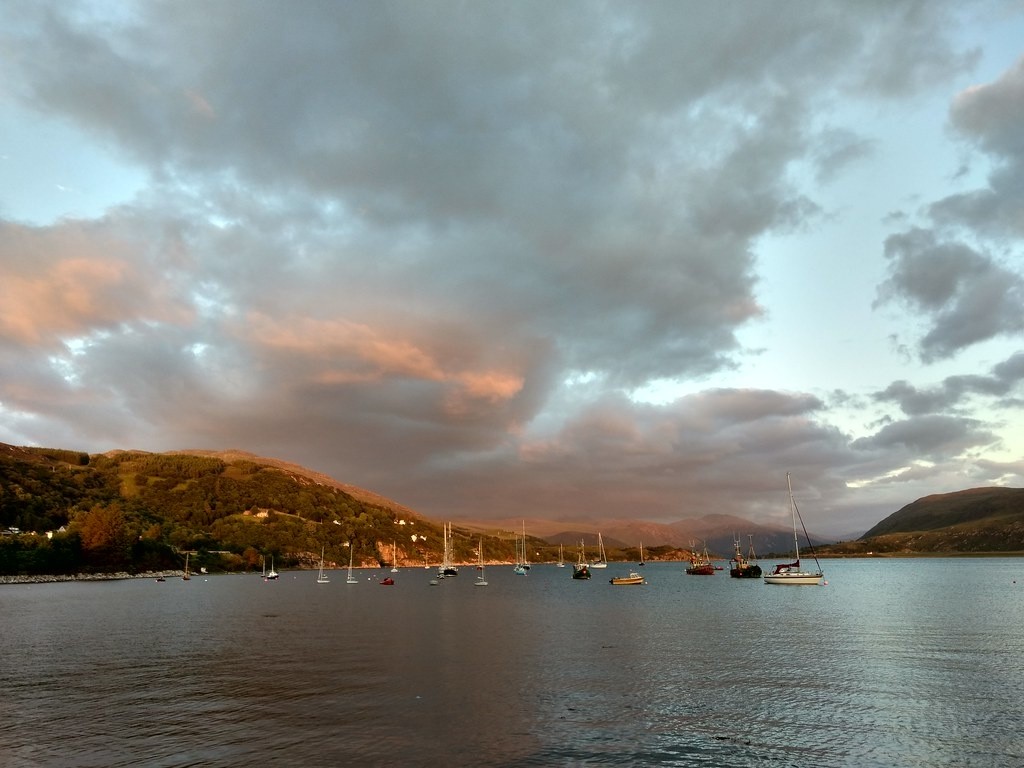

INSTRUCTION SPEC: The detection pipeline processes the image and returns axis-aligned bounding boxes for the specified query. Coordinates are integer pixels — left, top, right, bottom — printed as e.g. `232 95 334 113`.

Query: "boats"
156 578 166 582
609 572 644 586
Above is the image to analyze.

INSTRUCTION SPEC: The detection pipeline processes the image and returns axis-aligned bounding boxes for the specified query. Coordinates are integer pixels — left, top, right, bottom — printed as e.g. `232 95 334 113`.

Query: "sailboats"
684 539 723 575
260 521 488 587
638 540 646 566
763 470 825 585
183 553 191 580
556 530 609 580
514 520 531 575
729 534 762 579
